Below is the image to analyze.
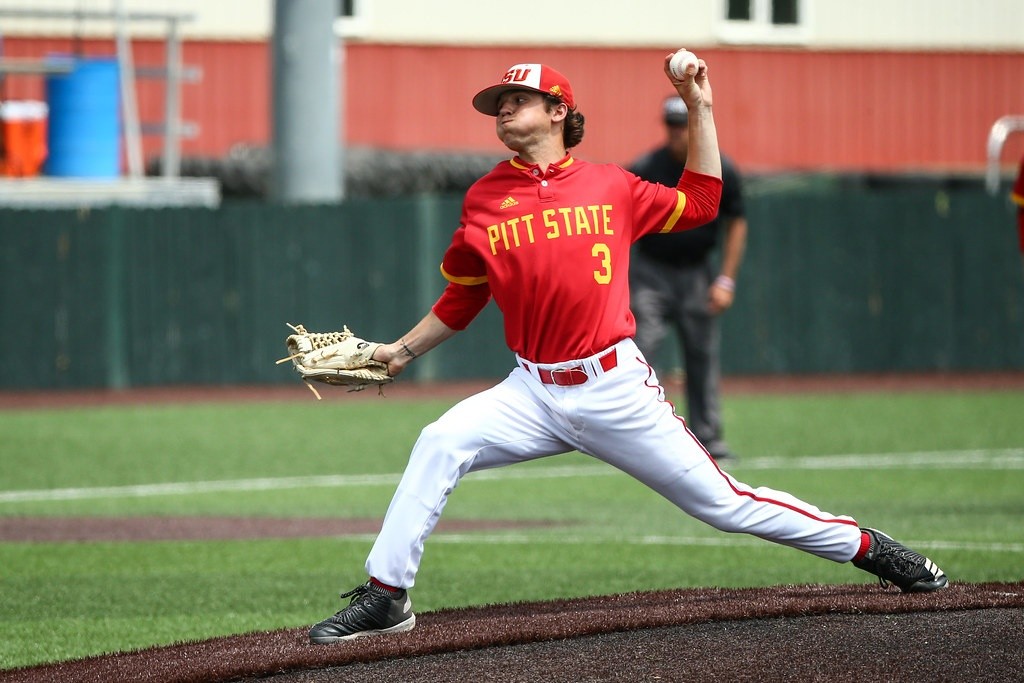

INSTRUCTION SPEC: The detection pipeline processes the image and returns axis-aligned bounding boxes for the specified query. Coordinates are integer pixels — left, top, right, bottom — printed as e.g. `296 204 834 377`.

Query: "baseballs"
670 51 700 81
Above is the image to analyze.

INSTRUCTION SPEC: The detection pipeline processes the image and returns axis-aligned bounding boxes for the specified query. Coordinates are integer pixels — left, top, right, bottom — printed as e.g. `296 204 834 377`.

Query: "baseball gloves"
284 330 395 388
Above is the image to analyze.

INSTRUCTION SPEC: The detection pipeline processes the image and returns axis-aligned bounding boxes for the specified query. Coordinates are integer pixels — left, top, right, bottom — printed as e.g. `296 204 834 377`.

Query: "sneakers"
308 583 416 645
852 529 950 594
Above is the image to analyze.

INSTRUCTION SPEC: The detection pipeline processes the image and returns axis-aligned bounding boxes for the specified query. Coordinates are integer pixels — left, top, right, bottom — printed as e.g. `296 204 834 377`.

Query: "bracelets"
715 275 736 292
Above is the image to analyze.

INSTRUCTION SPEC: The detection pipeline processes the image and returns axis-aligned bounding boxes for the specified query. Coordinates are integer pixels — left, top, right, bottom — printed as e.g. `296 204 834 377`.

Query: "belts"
522 349 619 386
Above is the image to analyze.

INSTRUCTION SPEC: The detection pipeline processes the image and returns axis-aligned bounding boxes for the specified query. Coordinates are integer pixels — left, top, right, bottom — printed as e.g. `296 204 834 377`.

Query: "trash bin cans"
40 55 120 179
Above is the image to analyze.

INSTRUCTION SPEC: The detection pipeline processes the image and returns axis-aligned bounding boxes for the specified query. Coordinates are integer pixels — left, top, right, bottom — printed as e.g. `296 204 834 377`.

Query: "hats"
472 63 574 116
665 97 690 127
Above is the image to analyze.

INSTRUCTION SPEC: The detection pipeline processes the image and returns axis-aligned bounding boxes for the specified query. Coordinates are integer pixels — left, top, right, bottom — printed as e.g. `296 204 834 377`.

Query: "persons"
285 48 949 643
623 95 748 462
